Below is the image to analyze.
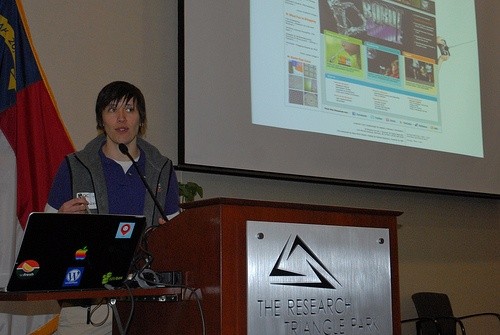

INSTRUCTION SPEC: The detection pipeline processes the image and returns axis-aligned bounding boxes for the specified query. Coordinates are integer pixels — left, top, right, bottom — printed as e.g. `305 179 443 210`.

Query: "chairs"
400 292 500 335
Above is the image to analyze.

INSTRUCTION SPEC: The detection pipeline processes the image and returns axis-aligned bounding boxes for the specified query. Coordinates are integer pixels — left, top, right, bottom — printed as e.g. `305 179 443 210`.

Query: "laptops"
0 212 146 294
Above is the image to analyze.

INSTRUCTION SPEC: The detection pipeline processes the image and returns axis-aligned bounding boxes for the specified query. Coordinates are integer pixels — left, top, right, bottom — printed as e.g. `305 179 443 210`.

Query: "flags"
0 0 78 230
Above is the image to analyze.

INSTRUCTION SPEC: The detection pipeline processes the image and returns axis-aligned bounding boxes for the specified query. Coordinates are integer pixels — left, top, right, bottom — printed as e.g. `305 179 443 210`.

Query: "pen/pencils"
78 193 91 214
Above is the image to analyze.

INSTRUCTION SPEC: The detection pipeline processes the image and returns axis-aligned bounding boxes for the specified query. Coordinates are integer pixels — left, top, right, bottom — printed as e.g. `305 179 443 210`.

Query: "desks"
0 286 202 335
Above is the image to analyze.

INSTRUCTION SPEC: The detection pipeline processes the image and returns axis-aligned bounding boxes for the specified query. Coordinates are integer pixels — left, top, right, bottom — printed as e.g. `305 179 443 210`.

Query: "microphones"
119 143 170 222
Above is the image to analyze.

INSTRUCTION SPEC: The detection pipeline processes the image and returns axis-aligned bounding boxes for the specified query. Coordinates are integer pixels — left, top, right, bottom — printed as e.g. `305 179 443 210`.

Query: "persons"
46 81 181 263
341 41 361 70
